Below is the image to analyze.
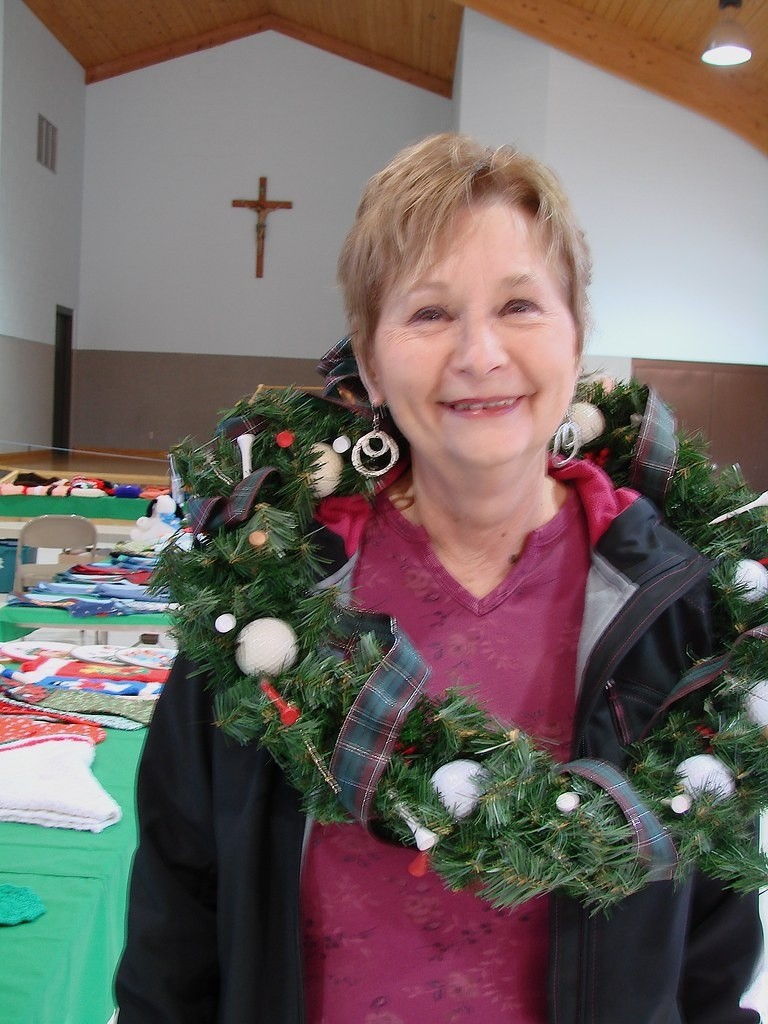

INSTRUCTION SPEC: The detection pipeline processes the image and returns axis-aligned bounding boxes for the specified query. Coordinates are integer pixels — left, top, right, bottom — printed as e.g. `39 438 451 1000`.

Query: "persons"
108 130 768 1024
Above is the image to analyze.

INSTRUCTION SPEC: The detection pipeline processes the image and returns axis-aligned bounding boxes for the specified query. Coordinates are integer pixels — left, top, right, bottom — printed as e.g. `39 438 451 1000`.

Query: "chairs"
13 514 98 599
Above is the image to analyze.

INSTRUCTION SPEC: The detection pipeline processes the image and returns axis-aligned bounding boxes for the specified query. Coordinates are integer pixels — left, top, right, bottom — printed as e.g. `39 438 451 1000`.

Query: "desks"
0 540 180 1024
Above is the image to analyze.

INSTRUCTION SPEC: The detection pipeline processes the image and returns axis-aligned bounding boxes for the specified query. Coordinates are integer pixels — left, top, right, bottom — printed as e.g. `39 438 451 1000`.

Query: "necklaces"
148 368 768 904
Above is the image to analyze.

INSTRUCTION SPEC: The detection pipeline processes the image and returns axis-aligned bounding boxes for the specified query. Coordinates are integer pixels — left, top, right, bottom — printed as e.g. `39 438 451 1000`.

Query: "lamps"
701 0 753 66
